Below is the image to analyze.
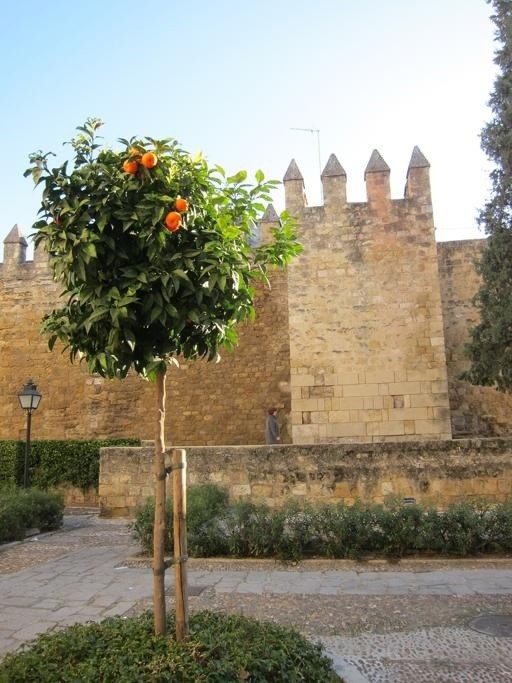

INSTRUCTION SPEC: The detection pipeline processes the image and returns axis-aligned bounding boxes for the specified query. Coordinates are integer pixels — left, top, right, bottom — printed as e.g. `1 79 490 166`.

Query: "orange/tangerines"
165 211 182 232
123 159 138 174
142 152 157 169
176 198 188 211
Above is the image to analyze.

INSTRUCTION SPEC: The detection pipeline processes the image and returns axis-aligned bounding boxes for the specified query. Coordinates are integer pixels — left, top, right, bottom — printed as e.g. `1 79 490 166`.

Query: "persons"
265 407 283 444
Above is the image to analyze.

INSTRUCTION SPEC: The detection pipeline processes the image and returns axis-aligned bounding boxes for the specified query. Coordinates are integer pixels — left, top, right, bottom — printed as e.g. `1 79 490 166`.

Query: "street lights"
18 377 42 490
